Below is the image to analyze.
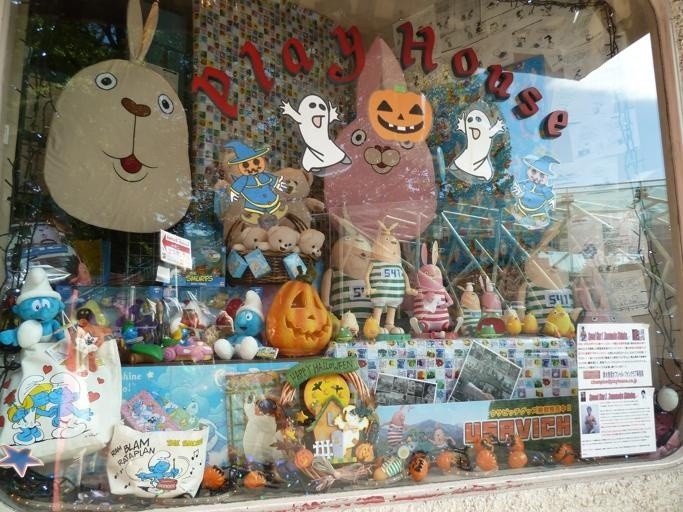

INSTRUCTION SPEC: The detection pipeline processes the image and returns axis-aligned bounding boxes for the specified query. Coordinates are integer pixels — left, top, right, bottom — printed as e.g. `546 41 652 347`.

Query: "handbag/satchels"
105 414 209 501
0 336 122 466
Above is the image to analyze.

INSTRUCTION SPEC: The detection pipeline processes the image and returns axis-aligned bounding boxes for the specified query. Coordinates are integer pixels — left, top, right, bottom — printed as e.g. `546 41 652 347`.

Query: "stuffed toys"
2 149 683 499
